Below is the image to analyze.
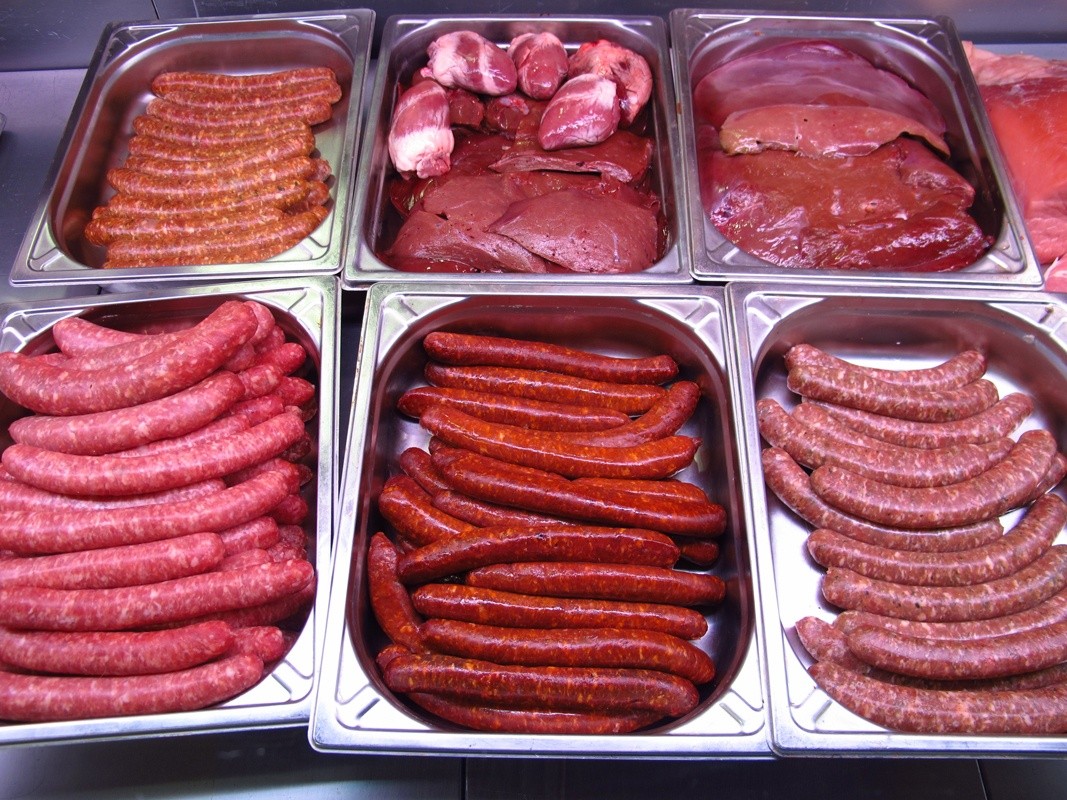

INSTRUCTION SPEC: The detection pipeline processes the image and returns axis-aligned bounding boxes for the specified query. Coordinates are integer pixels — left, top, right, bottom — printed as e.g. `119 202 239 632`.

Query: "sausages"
363 328 729 735
86 69 341 272
2 304 317 724
761 337 1066 735
